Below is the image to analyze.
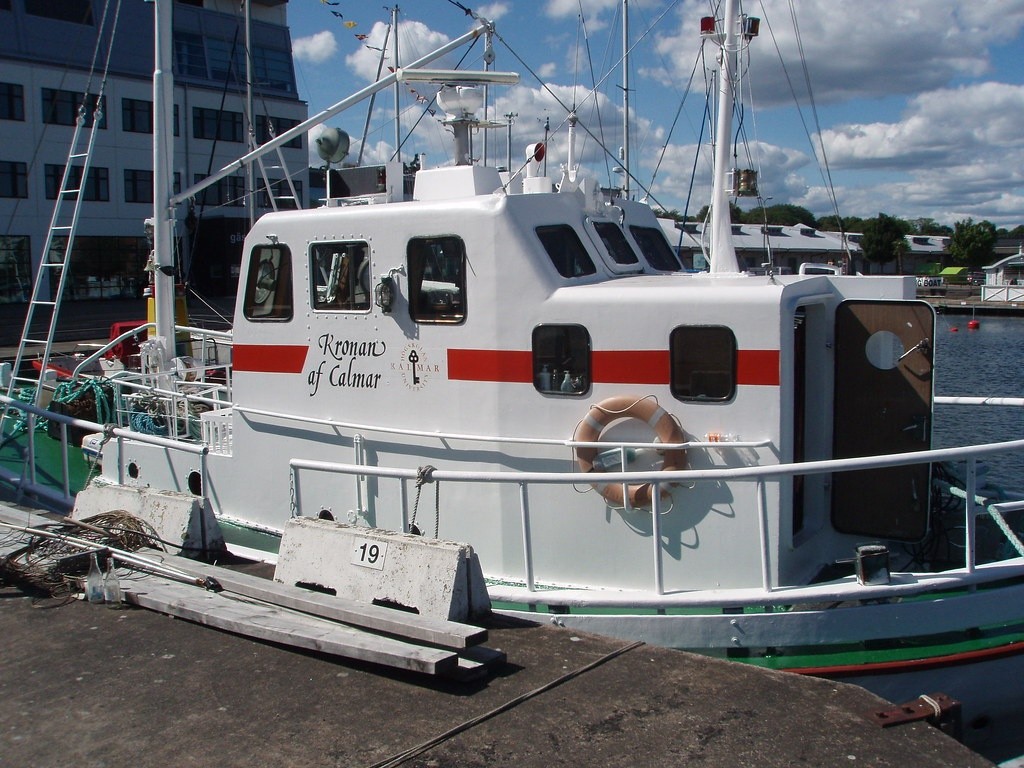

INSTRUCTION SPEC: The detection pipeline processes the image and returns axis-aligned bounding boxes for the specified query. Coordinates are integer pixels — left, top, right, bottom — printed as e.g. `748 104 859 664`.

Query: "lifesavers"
576 394 687 507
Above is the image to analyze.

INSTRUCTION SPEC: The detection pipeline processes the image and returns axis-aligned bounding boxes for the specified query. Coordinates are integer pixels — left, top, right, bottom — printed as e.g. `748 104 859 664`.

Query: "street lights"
755 196 774 260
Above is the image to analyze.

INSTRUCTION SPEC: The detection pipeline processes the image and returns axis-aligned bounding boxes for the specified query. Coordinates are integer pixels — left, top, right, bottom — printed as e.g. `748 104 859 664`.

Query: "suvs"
967 271 986 283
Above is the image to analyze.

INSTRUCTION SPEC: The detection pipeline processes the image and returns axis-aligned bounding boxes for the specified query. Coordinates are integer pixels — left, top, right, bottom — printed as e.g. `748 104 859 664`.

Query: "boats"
1 0 1024 723
